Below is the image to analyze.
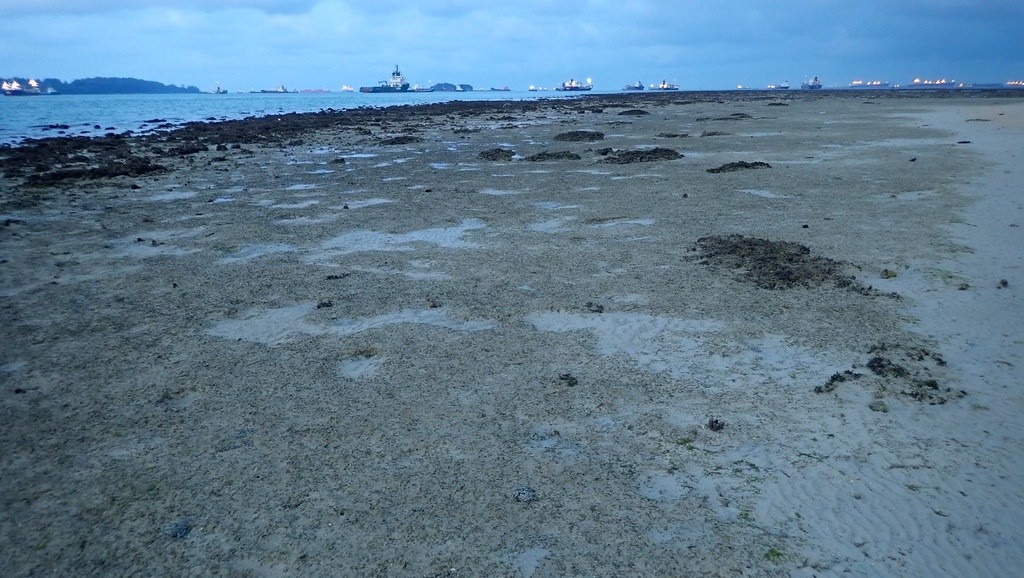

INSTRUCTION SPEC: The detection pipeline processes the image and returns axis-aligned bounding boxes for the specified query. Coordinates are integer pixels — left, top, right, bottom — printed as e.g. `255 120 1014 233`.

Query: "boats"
768 81 791 91
342 85 354 92
649 81 682 91
490 86 510 92
737 84 750 89
623 80 644 92
529 77 595 91
800 76 822 91
300 87 330 94
361 64 411 93
214 86 227 94
237 86 300 93
